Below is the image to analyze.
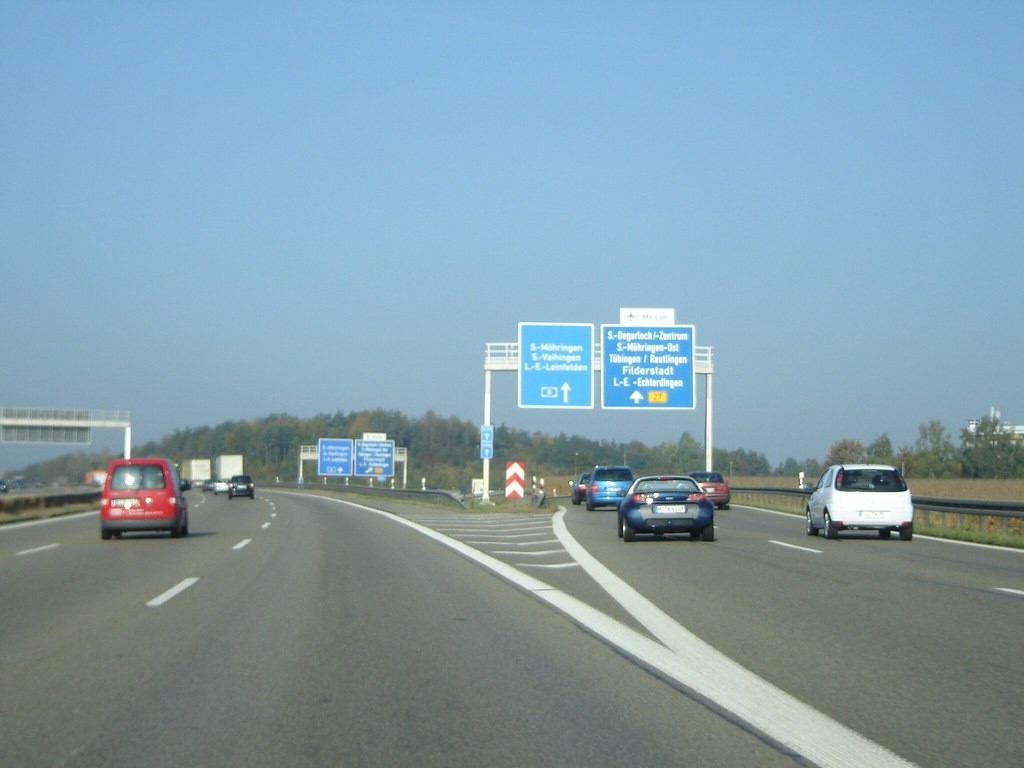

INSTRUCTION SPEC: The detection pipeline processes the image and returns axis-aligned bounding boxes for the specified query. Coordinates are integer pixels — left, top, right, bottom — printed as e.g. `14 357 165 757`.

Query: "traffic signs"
354 432 395 477
318 438 355 476
601 307 696 411
517 322 596 409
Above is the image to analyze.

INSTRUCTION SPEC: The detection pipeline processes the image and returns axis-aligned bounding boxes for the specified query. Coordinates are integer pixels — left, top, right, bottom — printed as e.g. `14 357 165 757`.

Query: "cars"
569 472 591 506
179 474 255 500
99 458 188 540
585 465 636 511
688 472 730 510
805 463 914 541
617 474 715 542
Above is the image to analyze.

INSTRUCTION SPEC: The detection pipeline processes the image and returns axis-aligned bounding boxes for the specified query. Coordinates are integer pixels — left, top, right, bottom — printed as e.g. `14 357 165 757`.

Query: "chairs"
872 475 880 486
